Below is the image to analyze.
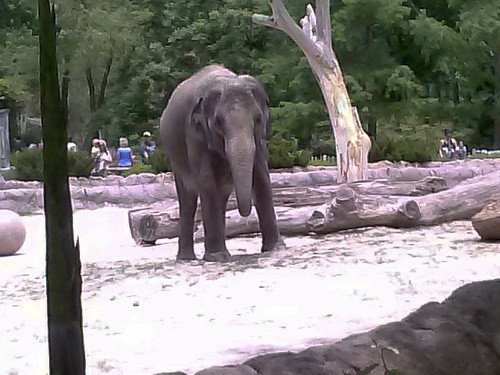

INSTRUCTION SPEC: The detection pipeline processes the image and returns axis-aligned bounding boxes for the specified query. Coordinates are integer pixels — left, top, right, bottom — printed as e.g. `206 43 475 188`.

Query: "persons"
12 131 156 176
438 129 467 159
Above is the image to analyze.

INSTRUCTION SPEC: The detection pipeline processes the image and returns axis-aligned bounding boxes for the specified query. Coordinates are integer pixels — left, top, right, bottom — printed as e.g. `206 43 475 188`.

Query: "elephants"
159 64 287 262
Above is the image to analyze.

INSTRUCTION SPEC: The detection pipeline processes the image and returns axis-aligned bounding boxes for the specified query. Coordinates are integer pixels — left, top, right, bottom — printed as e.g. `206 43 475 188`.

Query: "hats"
143 132 151 137
